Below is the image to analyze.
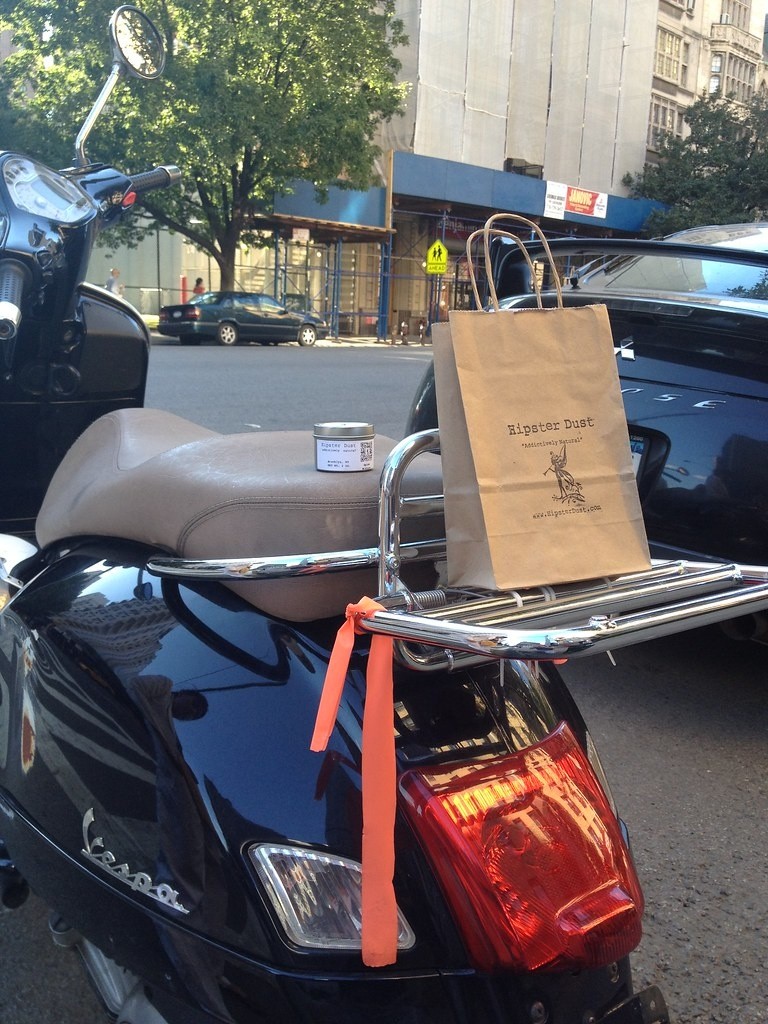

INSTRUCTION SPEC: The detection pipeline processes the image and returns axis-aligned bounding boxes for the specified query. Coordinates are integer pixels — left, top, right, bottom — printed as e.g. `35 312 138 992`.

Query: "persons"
105 267 126 297
193 277 208 296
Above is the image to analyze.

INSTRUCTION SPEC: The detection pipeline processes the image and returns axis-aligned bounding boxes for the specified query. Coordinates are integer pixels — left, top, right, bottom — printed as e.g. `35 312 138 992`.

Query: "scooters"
1 2 768 1024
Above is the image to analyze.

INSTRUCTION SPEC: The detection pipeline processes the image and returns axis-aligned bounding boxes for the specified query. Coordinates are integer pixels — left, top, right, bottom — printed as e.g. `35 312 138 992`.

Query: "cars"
156 289 329 347
401 214 768 656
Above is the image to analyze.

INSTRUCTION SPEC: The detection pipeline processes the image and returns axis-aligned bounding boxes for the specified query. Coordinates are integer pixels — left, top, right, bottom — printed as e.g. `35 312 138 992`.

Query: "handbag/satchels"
431 212 652 591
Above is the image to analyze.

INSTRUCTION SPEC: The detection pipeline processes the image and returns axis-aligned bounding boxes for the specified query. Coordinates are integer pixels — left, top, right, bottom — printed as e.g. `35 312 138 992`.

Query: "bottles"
313 421 375 473
